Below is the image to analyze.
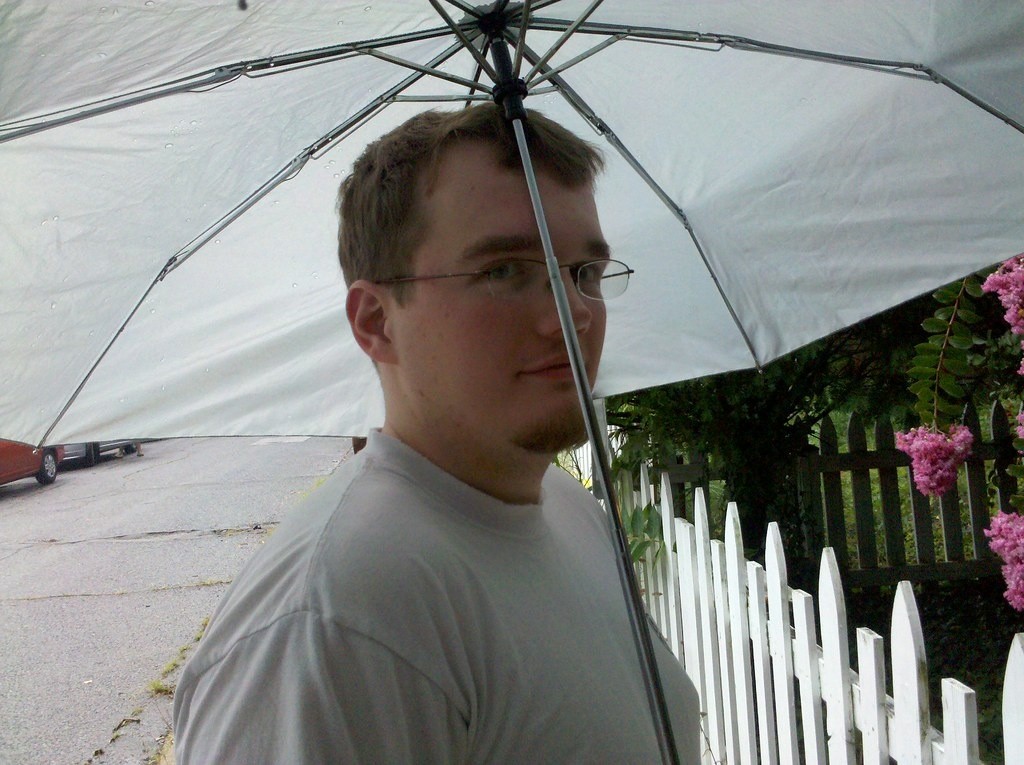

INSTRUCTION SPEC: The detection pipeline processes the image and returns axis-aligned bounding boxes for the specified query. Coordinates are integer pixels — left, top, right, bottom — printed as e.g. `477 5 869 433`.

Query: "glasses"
374 258 634 300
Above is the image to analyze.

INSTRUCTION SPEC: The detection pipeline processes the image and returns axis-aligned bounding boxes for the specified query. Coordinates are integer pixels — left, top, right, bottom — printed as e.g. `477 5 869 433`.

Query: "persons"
176 102 698 765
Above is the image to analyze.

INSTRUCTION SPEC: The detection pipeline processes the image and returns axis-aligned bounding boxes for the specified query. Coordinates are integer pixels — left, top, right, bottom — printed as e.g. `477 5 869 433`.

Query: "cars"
0 438 65 487
63 440 138 466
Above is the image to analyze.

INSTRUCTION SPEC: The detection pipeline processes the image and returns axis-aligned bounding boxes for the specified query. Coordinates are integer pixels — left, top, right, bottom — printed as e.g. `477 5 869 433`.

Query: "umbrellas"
0 0 1024 765
